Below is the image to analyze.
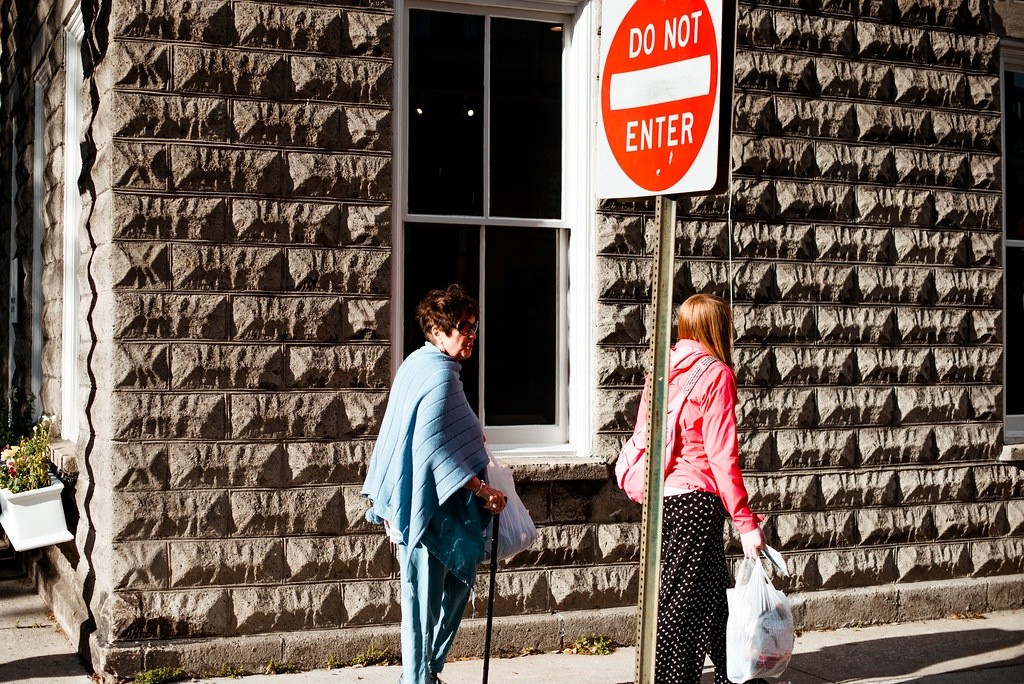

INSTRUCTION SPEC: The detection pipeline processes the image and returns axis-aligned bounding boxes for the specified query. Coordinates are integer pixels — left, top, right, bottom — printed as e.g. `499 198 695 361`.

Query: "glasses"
451 320 478 336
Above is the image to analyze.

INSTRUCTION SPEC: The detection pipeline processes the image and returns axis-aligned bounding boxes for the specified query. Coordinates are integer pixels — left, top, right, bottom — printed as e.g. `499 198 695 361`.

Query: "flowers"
0 413 60 493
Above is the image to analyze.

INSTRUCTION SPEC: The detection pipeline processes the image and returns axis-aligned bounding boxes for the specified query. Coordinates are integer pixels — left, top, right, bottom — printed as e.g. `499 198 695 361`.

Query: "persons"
360 284 509 684
614 293 766 684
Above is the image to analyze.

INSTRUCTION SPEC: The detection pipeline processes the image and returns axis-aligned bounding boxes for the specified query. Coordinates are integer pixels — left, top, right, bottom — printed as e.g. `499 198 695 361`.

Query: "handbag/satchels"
480 442 539 565
726 557 794 684
615 403 684 504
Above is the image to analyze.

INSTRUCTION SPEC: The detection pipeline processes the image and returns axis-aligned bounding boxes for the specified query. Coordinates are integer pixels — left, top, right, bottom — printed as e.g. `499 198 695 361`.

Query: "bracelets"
476 478 486 495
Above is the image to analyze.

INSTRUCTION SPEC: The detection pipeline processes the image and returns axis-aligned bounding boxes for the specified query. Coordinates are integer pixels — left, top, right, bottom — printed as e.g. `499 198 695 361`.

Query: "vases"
0 471 74 552
